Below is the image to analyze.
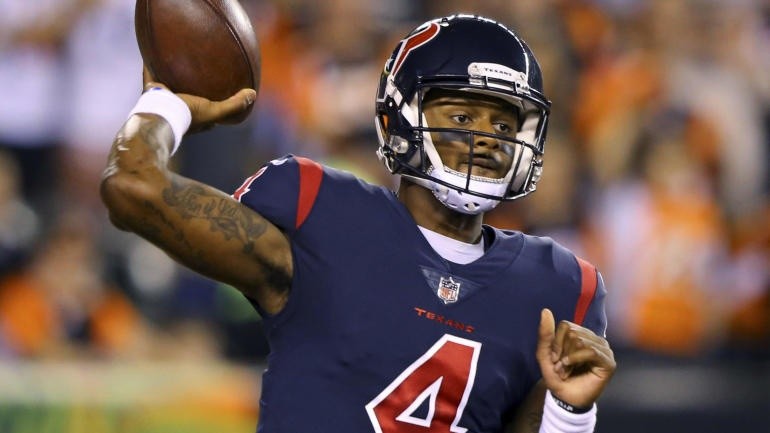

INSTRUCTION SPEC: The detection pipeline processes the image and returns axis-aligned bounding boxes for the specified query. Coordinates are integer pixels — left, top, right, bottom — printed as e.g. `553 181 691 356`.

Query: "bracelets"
552 393 592 414
128 87 192 157
537 389 597 433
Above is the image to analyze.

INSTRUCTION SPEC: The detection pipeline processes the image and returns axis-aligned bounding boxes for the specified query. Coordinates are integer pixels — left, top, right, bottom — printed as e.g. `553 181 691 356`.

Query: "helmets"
374 13 551 215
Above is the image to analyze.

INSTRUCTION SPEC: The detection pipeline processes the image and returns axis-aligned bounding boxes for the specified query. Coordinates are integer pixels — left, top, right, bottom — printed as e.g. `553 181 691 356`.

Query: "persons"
100 14 617 433
0 0 770 366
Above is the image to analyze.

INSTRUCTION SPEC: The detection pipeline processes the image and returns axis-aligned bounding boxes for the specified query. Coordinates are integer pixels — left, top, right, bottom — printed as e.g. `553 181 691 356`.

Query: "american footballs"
133 0 262 127
